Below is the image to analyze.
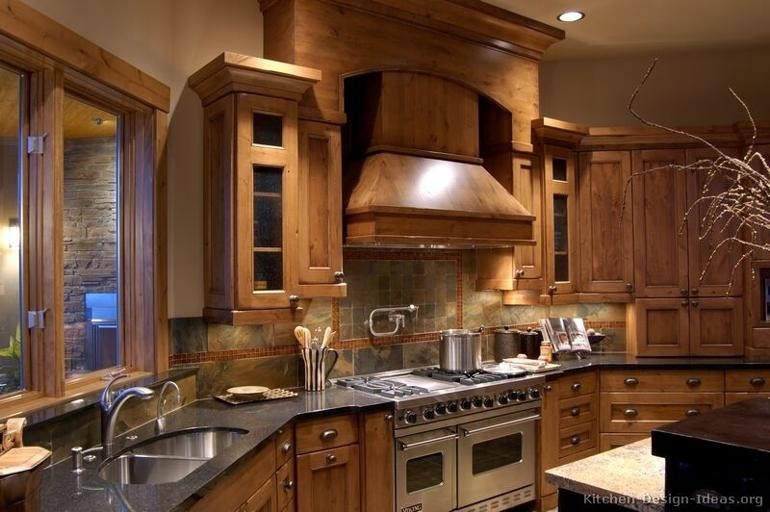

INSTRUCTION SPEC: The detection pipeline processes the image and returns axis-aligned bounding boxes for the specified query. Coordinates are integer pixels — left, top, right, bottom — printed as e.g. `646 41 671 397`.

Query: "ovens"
394 395 541 510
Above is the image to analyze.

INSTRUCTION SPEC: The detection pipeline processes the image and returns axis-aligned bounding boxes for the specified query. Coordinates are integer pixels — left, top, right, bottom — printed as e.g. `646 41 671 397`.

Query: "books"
539 316 592 354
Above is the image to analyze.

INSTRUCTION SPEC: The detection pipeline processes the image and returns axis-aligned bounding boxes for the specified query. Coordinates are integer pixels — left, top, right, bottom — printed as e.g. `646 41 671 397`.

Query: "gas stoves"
338 358 529 406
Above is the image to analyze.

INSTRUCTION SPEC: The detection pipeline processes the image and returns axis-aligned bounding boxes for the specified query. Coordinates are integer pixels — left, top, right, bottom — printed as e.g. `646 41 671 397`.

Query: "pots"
521 326 540 359
494 324 521 362
439 327 483 374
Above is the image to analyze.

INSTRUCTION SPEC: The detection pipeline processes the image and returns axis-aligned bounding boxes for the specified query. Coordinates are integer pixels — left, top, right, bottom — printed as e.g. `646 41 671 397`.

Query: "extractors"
342 96 537 254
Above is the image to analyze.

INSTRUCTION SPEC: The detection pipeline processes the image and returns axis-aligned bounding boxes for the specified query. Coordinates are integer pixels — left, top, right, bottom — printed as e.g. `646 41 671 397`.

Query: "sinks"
94 424 251 486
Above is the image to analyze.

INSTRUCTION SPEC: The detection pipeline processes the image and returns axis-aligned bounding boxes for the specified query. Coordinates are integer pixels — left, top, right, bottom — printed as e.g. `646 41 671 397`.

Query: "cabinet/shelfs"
297 117 346 294
537 365 770 512
625 295 745 359
175 403 395 512
500 117 588 308
187 52 321 326
632 126 743 297
735 122 770 262
477 152 545 292
577 127 633 304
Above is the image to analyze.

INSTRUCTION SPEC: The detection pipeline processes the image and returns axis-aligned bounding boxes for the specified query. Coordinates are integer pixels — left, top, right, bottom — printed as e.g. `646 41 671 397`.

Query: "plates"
225 385 269 401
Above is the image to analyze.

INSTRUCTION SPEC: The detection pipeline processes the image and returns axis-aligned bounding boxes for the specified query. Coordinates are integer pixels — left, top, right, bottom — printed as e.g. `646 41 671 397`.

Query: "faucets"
156 380 183 432
98 373 155 447
